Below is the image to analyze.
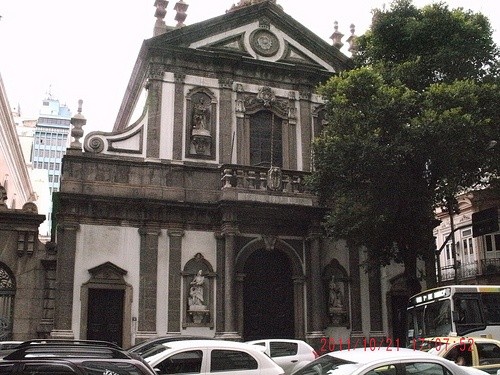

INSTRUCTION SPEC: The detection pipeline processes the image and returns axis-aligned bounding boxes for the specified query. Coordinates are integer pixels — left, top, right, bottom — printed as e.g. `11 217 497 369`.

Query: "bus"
396 281 500 346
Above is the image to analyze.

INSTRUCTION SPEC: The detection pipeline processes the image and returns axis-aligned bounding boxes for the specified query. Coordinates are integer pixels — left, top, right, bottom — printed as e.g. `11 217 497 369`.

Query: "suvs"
0 337 156 374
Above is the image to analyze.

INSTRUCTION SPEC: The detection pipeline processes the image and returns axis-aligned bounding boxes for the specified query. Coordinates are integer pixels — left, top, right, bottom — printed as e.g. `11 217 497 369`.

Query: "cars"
406 331 500 375
292 346 489 375
242 338 321 374
124 336 284 375
